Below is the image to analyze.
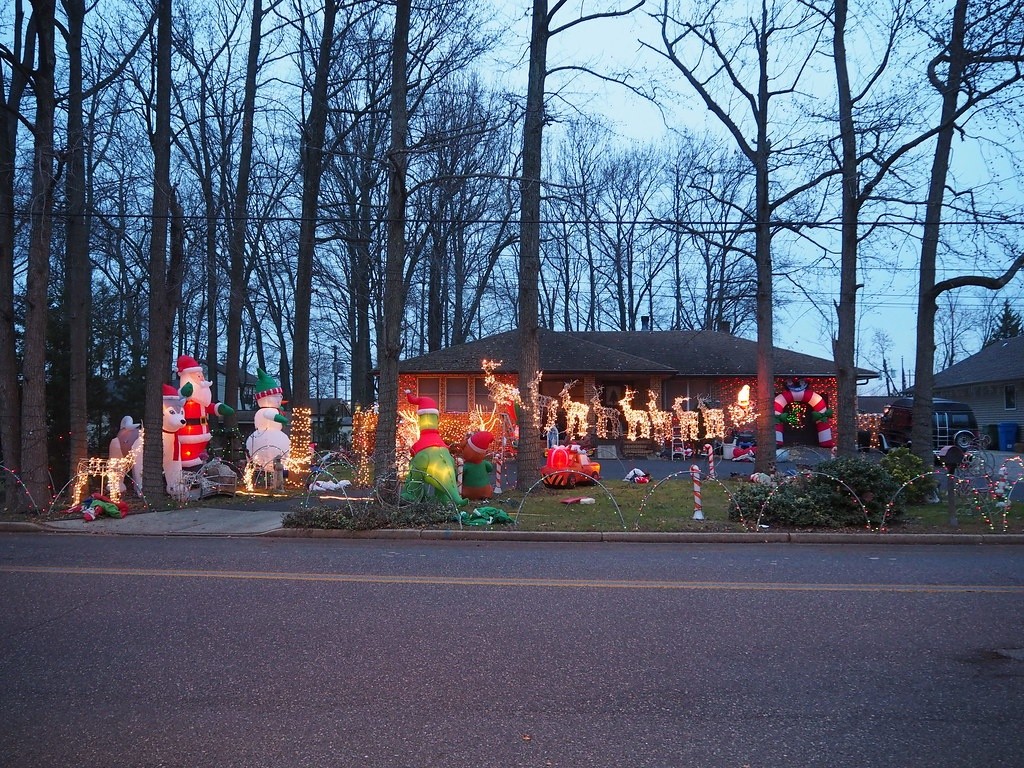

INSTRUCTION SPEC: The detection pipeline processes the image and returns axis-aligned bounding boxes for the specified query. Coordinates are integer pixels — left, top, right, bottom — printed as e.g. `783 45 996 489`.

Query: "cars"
542 444 601 485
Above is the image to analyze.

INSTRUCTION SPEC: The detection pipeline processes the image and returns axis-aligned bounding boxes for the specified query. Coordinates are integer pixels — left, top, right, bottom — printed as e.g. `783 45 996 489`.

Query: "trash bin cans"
998 421 1018 452
980 423 999 450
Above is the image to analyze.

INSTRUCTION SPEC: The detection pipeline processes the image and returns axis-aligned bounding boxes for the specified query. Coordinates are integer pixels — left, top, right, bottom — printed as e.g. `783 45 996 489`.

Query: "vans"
874 396 979 467
